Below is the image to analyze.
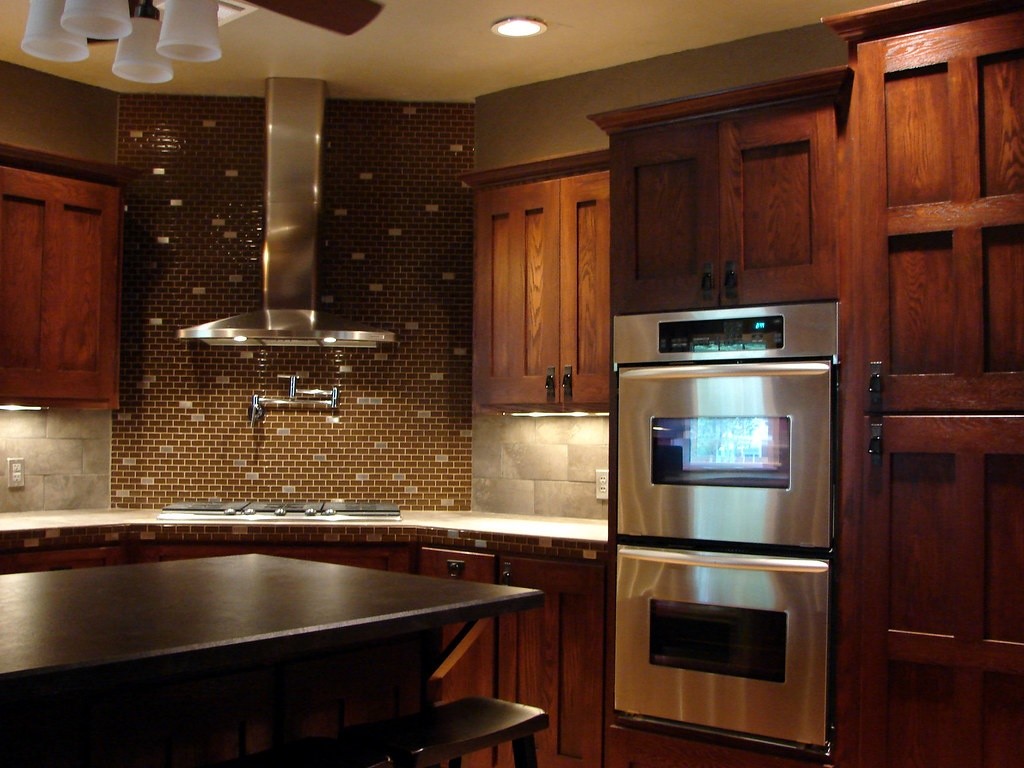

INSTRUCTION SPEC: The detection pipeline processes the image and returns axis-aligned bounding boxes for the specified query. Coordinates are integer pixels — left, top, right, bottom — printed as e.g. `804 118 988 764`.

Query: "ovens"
611 300 837 749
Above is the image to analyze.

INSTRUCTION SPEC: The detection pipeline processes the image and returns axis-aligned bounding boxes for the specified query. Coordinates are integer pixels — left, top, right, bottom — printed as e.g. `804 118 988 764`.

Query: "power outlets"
6 457 25 488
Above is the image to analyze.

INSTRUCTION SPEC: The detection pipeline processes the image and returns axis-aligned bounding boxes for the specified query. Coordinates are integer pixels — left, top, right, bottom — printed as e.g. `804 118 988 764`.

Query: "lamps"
21 0 223 85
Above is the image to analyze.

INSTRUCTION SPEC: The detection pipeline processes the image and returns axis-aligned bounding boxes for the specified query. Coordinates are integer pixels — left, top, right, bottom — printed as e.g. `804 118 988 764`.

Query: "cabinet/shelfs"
0 141 140 410
455 147 611 413
585 65 847 315
414 544 605 768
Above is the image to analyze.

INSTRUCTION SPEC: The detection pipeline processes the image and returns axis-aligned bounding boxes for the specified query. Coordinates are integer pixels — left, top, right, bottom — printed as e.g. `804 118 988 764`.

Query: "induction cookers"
156 501 402 522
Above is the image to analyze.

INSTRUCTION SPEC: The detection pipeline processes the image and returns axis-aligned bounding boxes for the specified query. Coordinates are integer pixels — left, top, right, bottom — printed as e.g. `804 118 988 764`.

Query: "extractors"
177 78 397 353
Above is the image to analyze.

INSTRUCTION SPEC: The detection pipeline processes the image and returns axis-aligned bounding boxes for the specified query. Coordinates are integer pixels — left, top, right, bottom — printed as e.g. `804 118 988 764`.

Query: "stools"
201 697 550 768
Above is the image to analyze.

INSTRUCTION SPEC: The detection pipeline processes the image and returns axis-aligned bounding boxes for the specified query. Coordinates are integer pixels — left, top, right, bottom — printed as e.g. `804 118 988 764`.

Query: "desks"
0 550 546 768
